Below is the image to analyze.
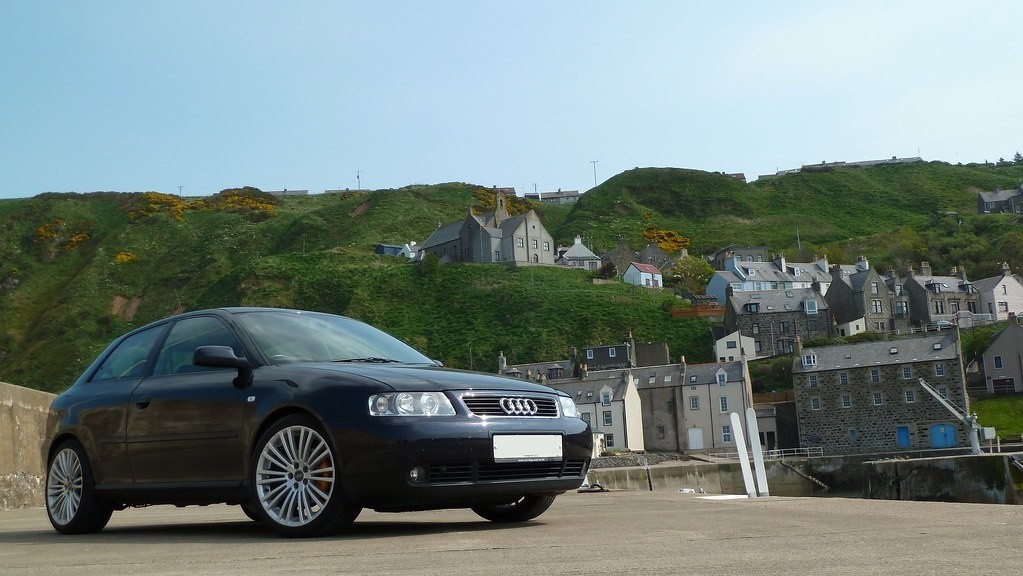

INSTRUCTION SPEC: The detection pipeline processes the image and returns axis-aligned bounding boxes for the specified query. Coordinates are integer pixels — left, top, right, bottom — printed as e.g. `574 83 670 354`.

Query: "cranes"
918 378 984 456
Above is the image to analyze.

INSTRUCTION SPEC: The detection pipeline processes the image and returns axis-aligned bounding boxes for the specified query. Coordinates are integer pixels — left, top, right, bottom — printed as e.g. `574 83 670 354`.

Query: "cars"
931 320 953 331
39 307 593 539
